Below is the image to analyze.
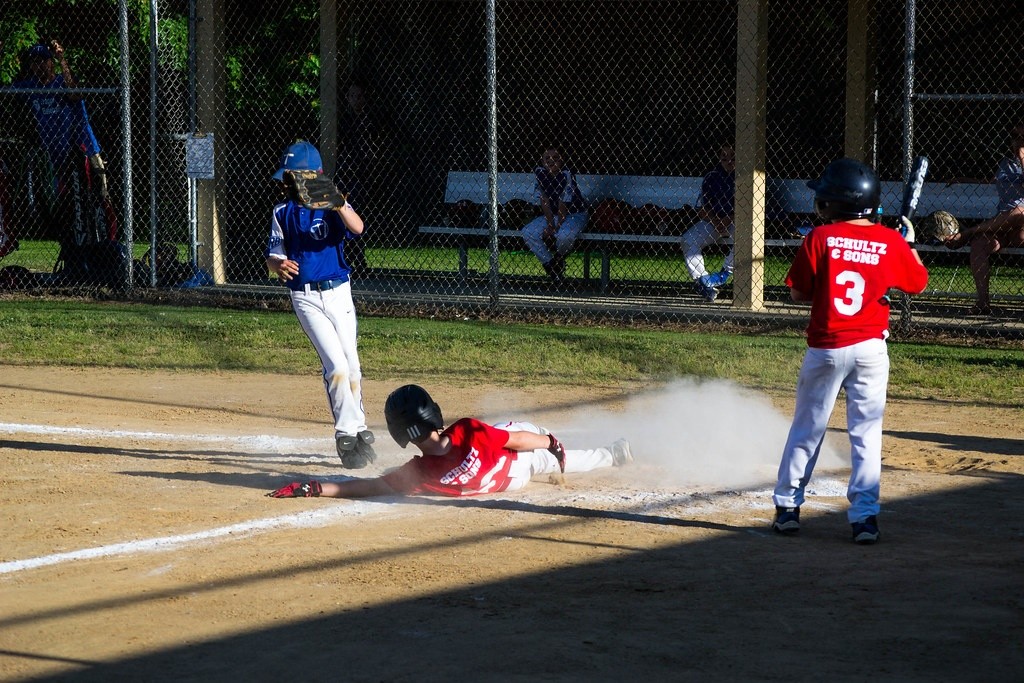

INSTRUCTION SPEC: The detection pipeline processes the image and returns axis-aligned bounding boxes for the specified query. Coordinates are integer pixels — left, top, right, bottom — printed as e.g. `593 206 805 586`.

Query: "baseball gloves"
282 169 345 211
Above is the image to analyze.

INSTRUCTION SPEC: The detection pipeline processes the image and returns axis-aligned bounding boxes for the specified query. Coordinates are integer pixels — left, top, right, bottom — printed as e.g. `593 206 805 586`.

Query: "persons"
944 129 1024 314
268 384 634 498
348 83 372 132
771 156 929 544
681 145 735 300
268 142 377 469
522 148 589 291
12 38 117 241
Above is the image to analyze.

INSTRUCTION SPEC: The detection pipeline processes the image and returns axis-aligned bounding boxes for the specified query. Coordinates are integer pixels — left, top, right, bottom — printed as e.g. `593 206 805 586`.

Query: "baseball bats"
898 155 930 237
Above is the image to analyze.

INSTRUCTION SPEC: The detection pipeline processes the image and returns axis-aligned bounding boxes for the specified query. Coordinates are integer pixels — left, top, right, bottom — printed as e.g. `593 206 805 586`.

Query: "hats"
272 142 322 179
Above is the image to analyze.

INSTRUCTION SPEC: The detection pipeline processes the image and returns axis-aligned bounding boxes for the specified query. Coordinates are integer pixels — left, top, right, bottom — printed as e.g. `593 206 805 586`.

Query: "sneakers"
851 515 879 543
357 431 377 463
772 504 800 530
336 436 366 469
605 438 634 466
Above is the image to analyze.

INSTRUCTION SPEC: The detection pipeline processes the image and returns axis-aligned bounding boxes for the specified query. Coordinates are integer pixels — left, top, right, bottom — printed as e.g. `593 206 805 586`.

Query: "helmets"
807 159 880 220
385 384 444 448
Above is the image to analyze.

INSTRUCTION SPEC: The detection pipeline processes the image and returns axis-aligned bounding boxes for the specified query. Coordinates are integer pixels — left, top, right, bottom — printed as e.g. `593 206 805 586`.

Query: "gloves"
895 215 915 244
547 434 566 473
269 480 322 498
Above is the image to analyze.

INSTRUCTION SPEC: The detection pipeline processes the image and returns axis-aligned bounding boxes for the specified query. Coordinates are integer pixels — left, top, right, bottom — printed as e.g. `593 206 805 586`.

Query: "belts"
297 276 348 292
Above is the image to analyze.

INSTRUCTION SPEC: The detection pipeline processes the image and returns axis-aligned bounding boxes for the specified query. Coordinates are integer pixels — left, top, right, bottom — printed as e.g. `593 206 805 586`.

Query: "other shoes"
539 270 576 293
694 282 718 301
701 269 733 287
939 235 962 250
966 303 990 315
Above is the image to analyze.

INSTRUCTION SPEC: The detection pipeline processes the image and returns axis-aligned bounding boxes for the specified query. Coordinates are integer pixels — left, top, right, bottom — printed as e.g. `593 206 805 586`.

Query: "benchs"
420 168 1024 297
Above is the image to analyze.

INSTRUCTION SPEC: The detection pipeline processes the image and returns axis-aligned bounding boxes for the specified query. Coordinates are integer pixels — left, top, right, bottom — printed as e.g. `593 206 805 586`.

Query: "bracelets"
908 242 916 249
